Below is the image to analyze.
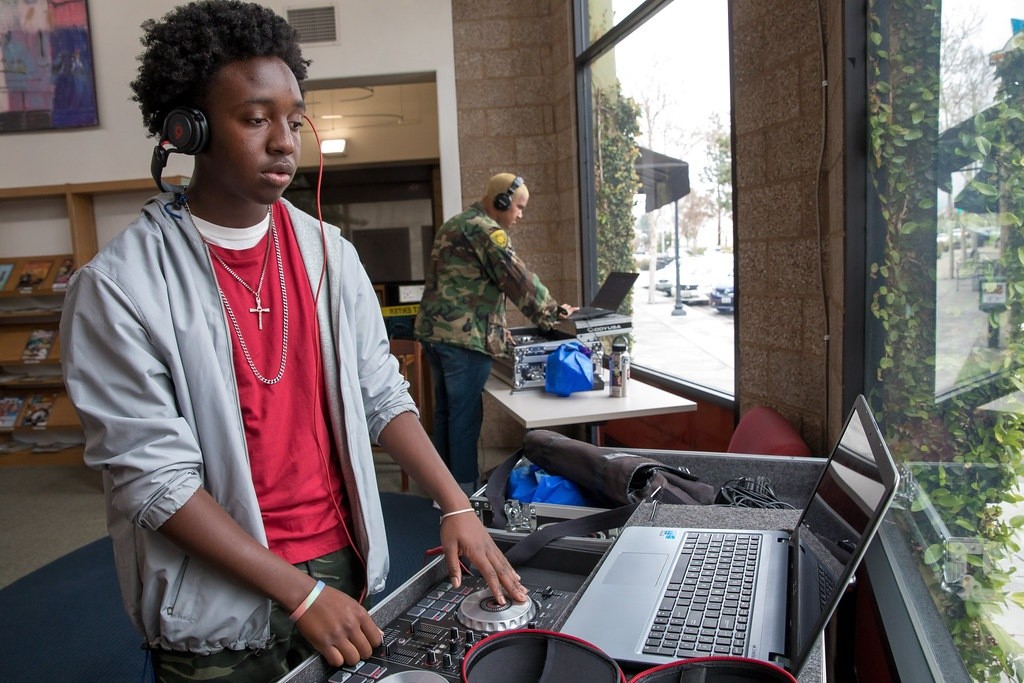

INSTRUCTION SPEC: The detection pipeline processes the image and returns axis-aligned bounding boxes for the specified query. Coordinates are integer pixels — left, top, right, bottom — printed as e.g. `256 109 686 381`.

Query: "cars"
655 257 701 295
711 282 735 314
936 227 973 262
671 253 734 306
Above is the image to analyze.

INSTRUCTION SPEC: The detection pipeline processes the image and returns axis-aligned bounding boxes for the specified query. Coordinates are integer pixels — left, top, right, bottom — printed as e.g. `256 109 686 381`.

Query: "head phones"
493 177 524 212
715 475 781 510
151 106 208 195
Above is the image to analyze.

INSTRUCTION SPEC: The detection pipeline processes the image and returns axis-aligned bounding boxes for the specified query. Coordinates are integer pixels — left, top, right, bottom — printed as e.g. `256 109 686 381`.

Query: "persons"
59 0 530 682
412 172 574 512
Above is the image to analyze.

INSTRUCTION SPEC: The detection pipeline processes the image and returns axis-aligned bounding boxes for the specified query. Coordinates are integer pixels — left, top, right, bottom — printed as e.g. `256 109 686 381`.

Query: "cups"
376 671 450 683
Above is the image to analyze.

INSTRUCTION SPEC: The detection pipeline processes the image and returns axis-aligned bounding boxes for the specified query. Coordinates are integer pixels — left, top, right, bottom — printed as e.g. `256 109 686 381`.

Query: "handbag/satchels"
545 341 593 398
519 428 714 512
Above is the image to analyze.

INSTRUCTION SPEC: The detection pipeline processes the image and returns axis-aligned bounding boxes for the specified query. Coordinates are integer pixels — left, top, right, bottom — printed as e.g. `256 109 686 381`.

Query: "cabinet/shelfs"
0 176 195 464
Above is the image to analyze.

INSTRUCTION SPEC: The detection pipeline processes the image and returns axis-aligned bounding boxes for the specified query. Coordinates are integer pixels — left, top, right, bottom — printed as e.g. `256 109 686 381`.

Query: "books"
0 392 58 431
0 259 74 291
0 441 74 454
22 326 58 360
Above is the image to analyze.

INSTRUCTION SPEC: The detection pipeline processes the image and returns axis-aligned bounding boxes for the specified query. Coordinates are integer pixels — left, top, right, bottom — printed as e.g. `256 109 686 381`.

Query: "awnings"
635 144 692 213
937 92 1015 212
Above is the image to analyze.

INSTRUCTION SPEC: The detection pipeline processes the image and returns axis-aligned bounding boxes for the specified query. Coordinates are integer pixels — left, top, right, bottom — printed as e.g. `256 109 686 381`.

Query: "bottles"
609 335 628 397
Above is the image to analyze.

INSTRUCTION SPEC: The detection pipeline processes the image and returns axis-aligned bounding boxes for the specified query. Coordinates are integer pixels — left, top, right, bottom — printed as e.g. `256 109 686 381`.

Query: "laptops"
558 393 900 682
566 272 640 321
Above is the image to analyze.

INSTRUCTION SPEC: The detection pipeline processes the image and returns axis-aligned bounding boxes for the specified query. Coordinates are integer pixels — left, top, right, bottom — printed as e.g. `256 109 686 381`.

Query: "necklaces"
182 189 274 331
221 211 289 384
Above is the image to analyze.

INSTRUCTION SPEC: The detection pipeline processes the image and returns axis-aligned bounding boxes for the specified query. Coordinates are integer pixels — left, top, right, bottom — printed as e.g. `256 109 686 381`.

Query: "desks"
482 367 696 448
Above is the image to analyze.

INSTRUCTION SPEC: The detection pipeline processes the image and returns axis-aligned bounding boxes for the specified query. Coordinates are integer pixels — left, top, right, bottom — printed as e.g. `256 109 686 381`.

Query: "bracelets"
289 579 325 624
439 508 475 524
557 306 568 320
